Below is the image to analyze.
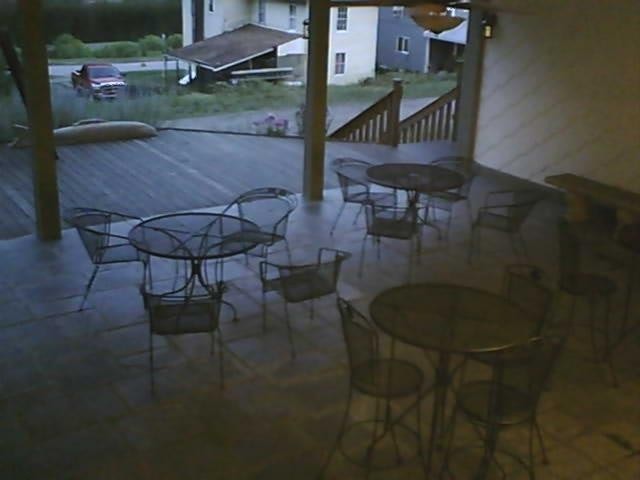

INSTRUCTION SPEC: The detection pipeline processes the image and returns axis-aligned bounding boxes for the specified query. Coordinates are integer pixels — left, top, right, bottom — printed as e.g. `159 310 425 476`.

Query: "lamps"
481 14 496 39
412 0 466 35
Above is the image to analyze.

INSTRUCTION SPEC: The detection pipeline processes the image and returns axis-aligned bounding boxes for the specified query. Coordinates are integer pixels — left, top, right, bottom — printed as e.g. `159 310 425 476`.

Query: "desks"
127 212 261 322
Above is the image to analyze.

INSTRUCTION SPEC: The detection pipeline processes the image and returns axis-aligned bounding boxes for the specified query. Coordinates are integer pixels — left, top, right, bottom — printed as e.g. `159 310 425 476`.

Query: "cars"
65 58 135 105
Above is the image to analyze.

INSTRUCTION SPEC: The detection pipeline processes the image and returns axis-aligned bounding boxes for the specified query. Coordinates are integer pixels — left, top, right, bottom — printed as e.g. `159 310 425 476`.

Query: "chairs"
258 247 353 360
139 280 227 403
61 207 153 311
218 186 300 282
